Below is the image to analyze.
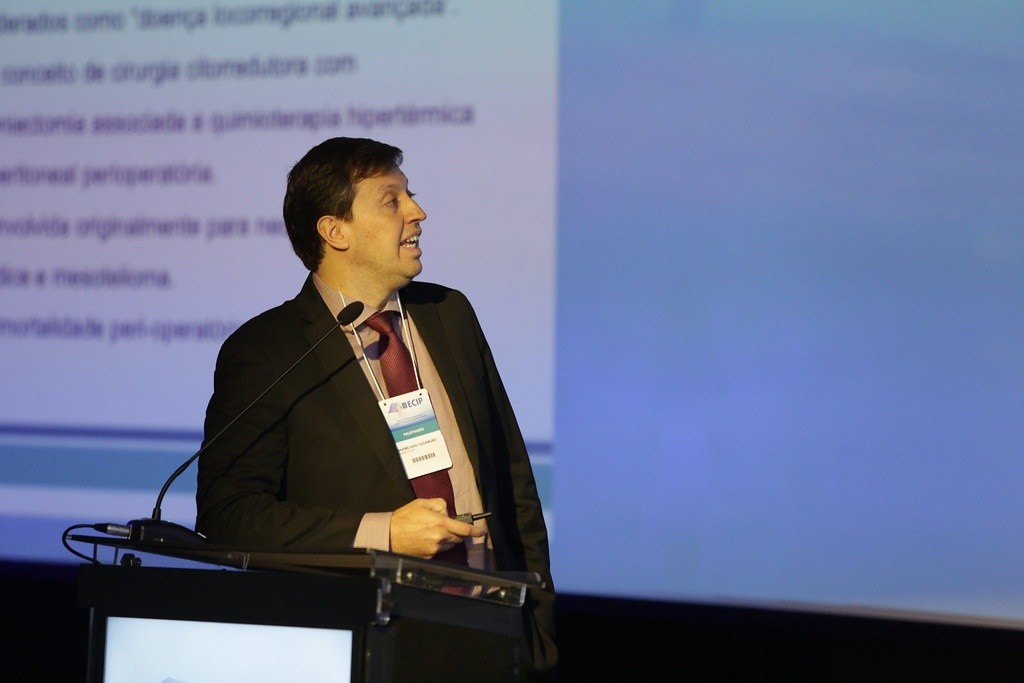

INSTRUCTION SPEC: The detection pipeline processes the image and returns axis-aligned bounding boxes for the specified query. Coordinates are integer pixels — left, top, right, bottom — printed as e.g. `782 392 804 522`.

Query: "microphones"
127 302 364 548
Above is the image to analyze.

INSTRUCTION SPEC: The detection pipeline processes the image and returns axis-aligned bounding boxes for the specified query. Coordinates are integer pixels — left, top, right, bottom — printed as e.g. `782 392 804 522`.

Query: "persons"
194 137 551 683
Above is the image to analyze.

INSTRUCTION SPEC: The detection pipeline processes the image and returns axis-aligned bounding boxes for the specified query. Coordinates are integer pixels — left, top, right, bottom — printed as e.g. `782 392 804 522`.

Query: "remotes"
451 512 492 525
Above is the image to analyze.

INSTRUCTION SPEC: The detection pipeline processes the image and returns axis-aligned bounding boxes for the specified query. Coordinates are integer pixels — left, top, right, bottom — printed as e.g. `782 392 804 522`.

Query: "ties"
364 310 458 522
